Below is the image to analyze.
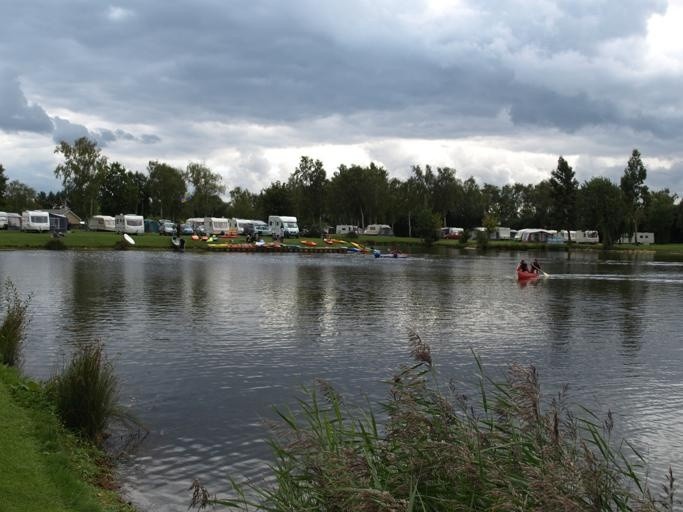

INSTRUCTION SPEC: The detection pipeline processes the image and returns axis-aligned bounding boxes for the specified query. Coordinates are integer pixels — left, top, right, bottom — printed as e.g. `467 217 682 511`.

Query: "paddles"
531 262 550 276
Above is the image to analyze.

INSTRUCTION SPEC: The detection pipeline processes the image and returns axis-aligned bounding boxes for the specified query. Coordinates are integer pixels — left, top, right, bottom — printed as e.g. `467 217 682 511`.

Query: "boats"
324 237 371 253
518 270 537 281
372 250 408 258
170 235 186 252
300 240 316 246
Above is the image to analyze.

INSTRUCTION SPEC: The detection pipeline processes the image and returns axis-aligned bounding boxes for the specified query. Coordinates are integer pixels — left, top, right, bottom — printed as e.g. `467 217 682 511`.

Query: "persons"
246 232 260 243
52 229 65 238
531 259 541 272
279 226 284 243
171 225 177 236
520 260 529 273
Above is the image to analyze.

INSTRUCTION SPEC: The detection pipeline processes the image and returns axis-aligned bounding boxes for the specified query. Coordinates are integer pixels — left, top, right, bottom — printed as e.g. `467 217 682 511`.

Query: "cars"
299 225 322 238
444 232 462 239
158 223 206 235
